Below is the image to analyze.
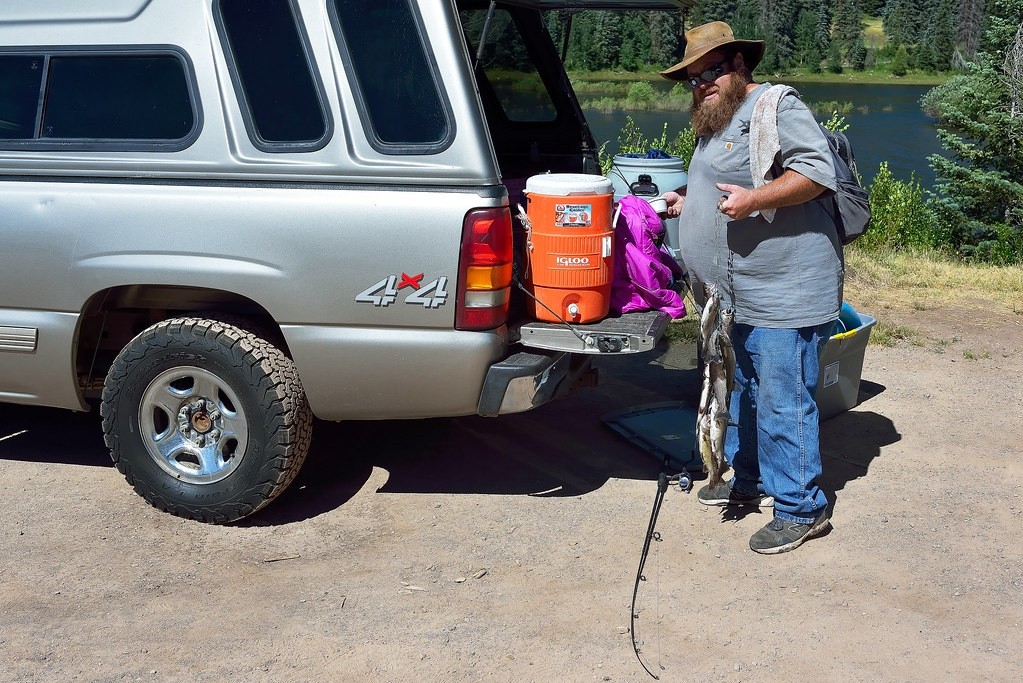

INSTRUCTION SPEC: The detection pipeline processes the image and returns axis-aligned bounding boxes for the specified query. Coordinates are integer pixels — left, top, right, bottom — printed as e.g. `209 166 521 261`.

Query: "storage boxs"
815 313 878 425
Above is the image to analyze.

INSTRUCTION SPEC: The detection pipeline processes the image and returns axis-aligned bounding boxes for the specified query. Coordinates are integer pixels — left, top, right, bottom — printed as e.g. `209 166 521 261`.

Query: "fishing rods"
630 458 693 680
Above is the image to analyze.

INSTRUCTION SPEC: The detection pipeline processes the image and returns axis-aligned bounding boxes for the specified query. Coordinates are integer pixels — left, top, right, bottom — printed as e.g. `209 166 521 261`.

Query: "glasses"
686 58 732 87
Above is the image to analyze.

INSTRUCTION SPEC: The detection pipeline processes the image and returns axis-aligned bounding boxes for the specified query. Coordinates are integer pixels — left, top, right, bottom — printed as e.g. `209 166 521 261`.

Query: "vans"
0 0 685 527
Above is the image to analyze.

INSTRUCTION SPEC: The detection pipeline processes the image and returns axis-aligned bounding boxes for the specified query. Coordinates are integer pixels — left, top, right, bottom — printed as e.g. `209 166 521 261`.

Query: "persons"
656 18 845 556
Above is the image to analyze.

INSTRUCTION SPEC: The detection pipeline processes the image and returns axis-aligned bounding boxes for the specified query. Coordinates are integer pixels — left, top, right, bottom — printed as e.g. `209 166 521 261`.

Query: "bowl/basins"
648 198 669 213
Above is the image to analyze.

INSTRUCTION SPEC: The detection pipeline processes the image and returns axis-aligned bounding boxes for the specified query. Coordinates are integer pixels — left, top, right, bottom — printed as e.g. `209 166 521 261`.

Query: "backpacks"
771 90 872 246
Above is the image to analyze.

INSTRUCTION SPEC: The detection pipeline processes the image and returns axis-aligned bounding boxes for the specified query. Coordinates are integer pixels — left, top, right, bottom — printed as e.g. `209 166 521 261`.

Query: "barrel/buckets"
519 171 615 325
605 154 690 276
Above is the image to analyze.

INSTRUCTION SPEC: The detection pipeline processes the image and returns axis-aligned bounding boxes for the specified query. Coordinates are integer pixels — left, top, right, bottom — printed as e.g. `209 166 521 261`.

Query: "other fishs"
696 293 736 490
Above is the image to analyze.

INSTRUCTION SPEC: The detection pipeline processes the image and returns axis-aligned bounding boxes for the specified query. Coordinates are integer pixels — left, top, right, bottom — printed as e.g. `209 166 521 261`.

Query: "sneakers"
696 479 775 507
748 511 828 555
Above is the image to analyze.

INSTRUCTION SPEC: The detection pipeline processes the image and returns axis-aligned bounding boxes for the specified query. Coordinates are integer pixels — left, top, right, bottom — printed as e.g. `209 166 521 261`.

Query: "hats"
659 20 766 82
